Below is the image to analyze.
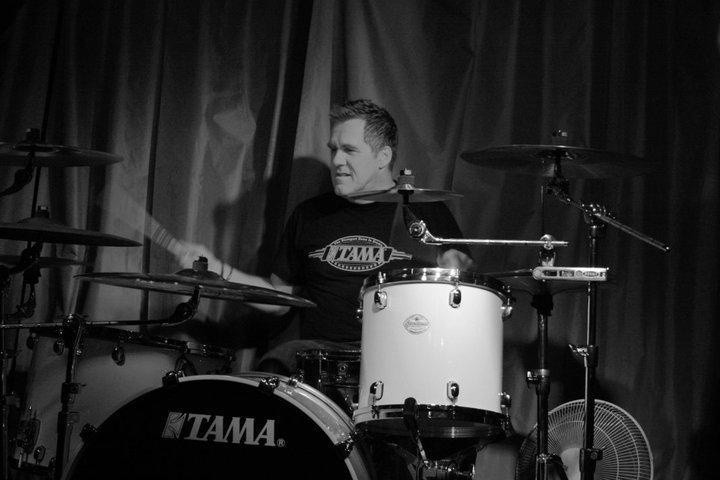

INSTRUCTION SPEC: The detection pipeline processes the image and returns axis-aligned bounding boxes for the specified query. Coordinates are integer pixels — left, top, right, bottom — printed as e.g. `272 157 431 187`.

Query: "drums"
354 269 512 438
13 325 236 473
67 372 373 479
296 351 360 388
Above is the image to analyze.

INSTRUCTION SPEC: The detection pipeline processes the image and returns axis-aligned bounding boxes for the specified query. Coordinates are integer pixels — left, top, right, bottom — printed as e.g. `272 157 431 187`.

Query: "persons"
166 99 476 379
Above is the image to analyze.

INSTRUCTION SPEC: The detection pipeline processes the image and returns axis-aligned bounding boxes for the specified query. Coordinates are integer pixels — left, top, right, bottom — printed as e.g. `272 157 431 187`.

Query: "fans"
517 398 655 479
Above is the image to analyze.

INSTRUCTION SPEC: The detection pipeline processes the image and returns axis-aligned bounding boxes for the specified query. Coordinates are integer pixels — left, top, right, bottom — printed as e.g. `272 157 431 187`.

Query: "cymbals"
72 272 317 308
349 191 464 202
463 146 659 179
2 223 142 246
0 145 122 167
2 255 86 265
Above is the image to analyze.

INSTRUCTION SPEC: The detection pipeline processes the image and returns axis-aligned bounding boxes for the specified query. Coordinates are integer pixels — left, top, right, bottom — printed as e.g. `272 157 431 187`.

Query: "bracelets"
223 265 235 283
217 261 226 278
